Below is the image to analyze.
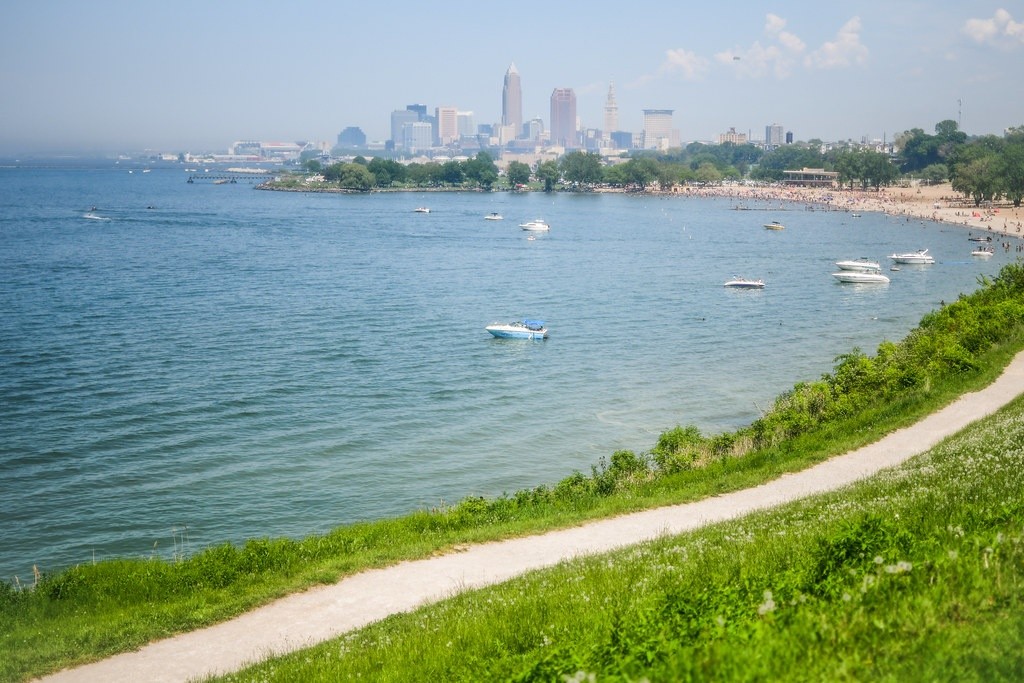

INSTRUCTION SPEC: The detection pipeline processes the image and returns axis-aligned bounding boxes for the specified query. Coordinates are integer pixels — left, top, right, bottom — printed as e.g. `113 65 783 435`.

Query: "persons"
657 186 1024 253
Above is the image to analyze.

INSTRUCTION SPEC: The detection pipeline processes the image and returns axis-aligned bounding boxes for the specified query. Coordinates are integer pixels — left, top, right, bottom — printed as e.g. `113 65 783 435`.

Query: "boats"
484 212 503 221
483 319 549 341
519 220 549 231
415 208 431 213
833 270 890 284
764 223 785 231
971 249 994 257
835 261 881 271
722 278 765 289
887 249 937 266
